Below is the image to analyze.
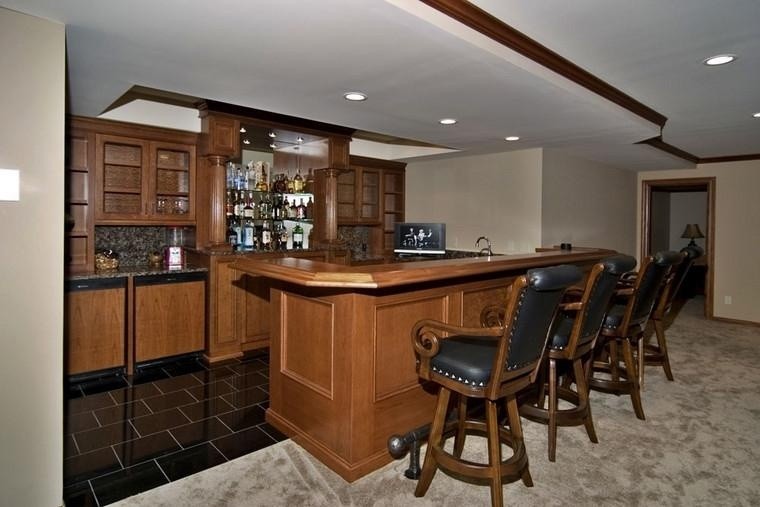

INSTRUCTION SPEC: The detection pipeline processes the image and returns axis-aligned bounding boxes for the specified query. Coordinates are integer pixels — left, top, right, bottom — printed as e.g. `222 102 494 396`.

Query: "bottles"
225 162 306 250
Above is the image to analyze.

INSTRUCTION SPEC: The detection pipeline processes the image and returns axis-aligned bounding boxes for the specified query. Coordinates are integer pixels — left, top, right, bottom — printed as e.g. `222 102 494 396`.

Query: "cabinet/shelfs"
224 175 318 252
335 153 407 255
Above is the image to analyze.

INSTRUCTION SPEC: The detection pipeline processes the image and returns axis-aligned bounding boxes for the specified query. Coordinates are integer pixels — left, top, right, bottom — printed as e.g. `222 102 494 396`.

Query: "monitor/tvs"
393 222 446 256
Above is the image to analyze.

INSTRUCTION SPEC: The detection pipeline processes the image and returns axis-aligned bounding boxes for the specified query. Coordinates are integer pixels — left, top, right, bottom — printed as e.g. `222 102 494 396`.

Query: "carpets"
95 293 759 506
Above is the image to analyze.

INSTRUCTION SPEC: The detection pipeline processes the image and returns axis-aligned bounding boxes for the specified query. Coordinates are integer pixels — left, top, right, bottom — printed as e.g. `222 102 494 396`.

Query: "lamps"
680 223 705 246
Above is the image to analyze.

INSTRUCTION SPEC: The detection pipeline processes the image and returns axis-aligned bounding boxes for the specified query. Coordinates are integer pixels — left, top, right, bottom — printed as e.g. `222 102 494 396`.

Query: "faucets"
474 236 492 256
